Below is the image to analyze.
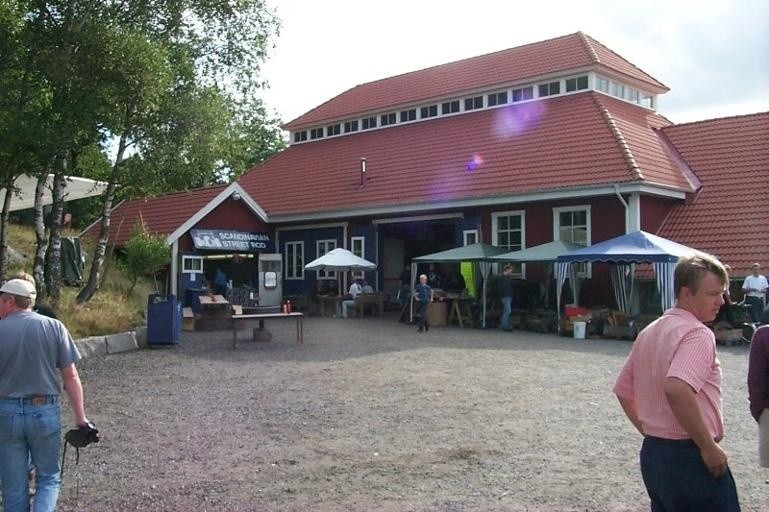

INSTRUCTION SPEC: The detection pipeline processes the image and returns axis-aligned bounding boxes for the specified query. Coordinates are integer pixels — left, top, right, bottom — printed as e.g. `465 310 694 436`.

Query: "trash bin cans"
146 294 183 345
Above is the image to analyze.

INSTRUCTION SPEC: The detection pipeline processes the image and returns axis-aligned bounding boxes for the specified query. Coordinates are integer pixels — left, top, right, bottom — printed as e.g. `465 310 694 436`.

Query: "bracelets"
758 288 761 292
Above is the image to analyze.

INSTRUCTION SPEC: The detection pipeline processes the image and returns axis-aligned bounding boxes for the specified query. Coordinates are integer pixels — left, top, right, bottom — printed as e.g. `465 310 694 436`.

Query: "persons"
214 268 227 298
7 271 57 318
740 263 769 323
312 264 515 332
0 279 88 511
613 253 741 511
748 325 769 470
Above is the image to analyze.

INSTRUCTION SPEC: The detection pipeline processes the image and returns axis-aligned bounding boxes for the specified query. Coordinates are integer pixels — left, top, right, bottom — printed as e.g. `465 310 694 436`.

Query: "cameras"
64 428 98 447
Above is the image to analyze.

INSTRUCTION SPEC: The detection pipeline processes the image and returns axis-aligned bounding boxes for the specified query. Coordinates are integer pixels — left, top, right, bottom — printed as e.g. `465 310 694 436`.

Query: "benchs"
349 293 384 318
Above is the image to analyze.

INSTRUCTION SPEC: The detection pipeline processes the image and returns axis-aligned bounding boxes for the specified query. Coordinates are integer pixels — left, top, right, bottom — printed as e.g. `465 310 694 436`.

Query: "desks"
231 312 304 351
430 294 476 328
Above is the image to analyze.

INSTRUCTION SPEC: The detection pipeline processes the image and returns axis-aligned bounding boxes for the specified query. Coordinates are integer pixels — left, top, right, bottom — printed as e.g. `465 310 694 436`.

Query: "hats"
0 278 37 300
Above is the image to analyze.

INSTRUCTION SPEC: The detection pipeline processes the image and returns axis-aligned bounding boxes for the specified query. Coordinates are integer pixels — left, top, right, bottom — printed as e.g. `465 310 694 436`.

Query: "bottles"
286 300 291 313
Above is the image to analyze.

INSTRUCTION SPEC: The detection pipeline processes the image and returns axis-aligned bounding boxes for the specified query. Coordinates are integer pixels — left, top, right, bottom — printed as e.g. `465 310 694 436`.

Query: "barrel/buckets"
574 322 586 338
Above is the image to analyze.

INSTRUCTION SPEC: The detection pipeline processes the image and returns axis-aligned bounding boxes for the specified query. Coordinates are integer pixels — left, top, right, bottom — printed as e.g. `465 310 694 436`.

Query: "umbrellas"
305 248 378 296
